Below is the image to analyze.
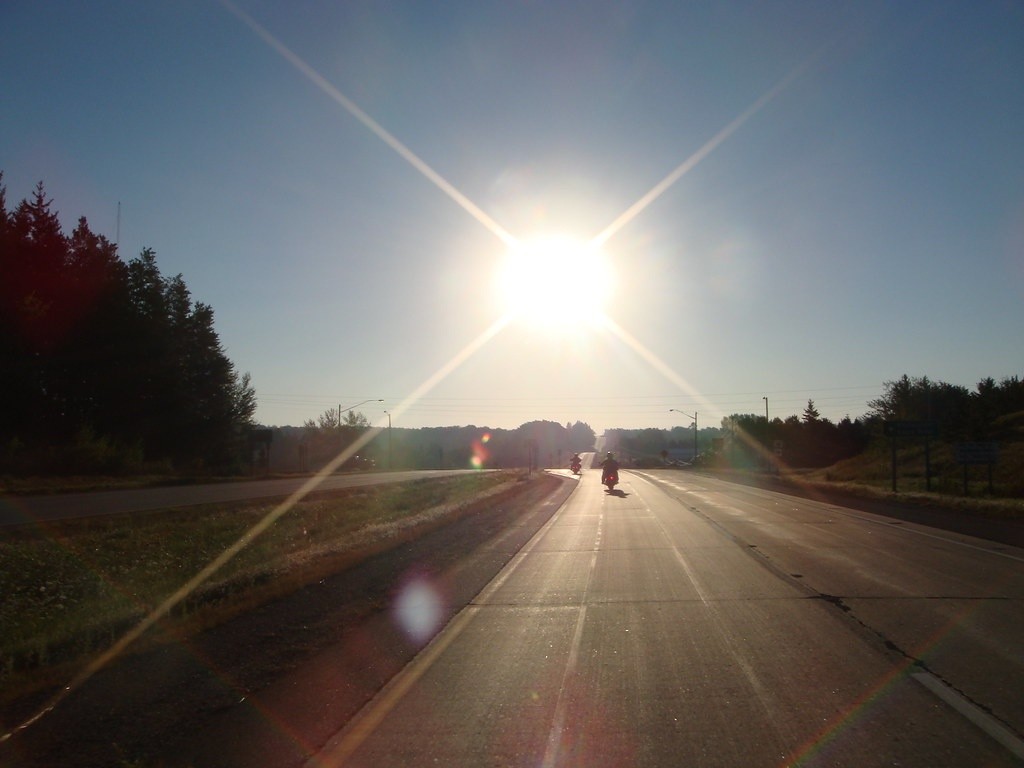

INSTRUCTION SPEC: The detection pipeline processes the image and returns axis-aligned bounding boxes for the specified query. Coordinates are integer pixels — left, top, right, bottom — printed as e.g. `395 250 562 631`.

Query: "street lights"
762 396 769 477
728 414 734 469
669 408 698 464
338 399 385 473
384 410 392 470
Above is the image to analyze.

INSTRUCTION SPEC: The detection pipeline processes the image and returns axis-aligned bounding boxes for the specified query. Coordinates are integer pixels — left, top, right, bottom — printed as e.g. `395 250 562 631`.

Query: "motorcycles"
569 458 582 474
597 462 619 491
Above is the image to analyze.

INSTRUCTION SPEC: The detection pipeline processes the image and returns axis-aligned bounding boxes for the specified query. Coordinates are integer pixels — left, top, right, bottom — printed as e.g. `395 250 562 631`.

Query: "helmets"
607 451 613 457
574 453 578 457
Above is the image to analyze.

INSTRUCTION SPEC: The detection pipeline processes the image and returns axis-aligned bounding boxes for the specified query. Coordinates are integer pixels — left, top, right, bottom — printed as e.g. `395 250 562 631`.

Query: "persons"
600 452 619 483
570 453 581 470
